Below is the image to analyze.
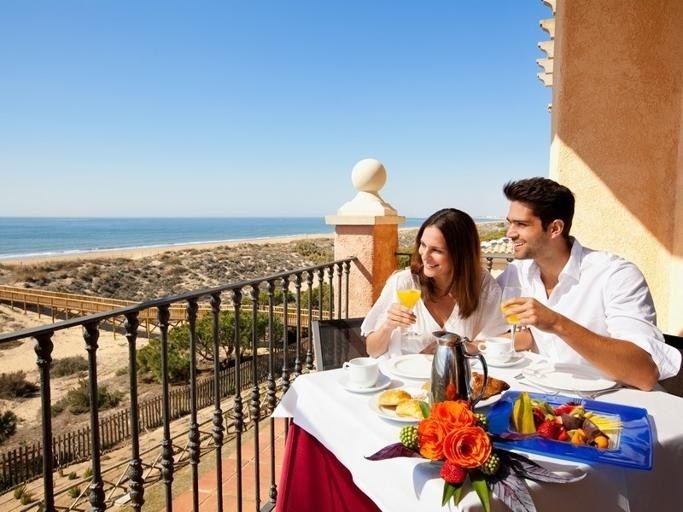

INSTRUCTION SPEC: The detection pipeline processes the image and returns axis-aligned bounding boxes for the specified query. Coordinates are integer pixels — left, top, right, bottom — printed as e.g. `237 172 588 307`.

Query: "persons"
493 177 682 391
359 208 533 357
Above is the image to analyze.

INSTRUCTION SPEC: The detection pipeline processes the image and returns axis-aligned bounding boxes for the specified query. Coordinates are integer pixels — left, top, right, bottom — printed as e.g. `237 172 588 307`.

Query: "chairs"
310 317 370 370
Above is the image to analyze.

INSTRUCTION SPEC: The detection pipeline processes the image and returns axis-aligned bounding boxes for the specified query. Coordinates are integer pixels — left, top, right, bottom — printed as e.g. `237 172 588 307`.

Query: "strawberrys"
537 419 557 440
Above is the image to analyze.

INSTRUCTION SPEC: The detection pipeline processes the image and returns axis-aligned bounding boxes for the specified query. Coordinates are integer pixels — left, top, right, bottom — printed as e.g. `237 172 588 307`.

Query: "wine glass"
500 286 530 356
396 274 422 337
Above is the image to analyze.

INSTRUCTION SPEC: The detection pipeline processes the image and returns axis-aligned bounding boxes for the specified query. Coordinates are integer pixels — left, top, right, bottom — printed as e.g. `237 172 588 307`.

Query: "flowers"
366 397 567 510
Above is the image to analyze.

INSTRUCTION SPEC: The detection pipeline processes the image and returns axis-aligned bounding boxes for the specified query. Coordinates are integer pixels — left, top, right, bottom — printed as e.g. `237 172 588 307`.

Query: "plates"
368 386 431 422
386 353 435 380
486 352 525 368
339 375 392 394
521 362 617 392
475 390 507 407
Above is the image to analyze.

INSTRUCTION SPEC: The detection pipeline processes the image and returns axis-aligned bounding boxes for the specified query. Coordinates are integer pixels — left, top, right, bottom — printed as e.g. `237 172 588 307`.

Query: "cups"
477 337 515 364
343 357 379 387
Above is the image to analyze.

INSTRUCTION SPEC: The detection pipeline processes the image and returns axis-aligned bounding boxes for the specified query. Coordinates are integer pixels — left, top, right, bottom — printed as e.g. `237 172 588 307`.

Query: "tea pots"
430 330 487 408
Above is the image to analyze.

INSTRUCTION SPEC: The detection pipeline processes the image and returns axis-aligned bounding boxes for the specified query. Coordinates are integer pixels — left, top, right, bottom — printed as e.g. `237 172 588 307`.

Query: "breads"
471 371 510 402
378 389 411 406
396 399 426 421
